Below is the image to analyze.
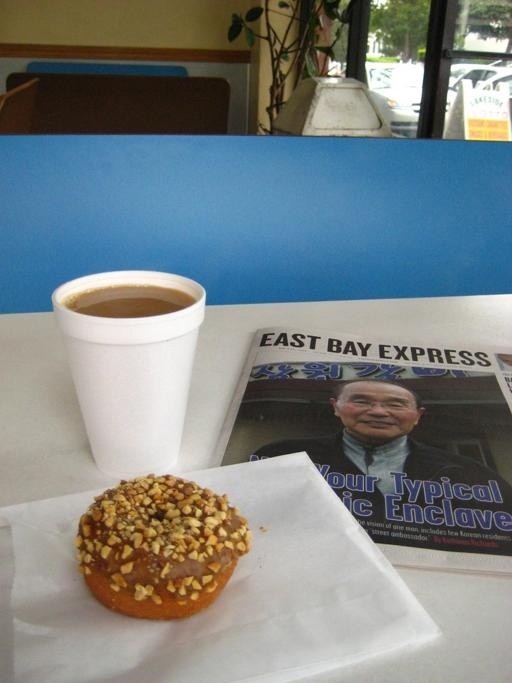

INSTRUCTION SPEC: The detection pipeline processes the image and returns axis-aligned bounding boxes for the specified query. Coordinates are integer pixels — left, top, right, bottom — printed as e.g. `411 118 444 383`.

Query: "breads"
75 476 244 616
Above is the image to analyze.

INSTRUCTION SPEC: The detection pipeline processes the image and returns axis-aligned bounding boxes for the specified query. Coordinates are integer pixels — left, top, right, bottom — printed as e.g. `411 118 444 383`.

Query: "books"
208 325 512 576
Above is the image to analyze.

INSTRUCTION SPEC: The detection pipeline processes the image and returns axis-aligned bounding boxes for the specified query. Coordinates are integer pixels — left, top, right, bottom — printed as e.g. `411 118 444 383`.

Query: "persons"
247 378 511 554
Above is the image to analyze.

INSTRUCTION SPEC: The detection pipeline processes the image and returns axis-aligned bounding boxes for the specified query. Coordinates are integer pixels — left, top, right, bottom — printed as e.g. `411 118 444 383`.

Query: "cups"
51 270 206 480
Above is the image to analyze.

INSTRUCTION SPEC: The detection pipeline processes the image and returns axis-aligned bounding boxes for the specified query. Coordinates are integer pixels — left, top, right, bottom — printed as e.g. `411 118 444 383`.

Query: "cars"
410 63 497 113
338 62 425 112
488 58 512 66
473 67 512 123
